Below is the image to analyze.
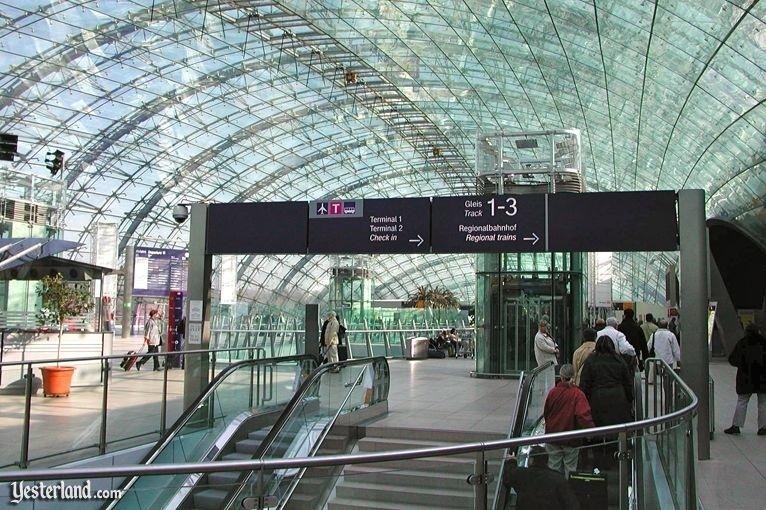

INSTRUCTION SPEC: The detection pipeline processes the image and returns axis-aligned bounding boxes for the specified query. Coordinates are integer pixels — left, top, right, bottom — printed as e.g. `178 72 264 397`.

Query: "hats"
582 328 596 340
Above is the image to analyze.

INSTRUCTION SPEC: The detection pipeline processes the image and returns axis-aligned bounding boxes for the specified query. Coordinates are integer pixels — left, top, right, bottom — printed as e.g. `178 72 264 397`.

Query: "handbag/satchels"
650 347 654 357
567 418 583 447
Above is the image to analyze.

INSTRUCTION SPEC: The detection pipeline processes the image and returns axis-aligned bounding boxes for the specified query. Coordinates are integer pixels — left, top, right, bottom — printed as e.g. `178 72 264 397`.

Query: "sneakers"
724 427 739 434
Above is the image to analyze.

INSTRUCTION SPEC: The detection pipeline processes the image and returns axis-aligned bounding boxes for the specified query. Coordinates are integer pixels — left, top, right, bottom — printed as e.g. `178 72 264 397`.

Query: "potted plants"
34 274 97 396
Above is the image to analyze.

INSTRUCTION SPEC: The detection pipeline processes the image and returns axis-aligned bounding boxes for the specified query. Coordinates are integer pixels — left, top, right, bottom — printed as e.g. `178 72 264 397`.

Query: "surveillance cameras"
172 206 188 223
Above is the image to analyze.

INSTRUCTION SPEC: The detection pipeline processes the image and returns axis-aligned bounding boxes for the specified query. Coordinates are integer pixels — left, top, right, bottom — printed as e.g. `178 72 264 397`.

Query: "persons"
724 322 766 436
136 309 164 372
501 446 581 510
252 311 285 337
320 312 341 373
533 318 560 401
436 328 462 358
573 307 681 465
543 364 596 481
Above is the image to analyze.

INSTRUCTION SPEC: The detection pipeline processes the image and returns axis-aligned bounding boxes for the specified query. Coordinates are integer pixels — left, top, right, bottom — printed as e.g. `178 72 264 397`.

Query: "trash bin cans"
405 336 429 360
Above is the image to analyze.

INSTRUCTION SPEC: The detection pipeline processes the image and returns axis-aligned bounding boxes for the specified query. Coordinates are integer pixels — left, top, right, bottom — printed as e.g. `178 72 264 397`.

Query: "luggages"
568 471 608 510
